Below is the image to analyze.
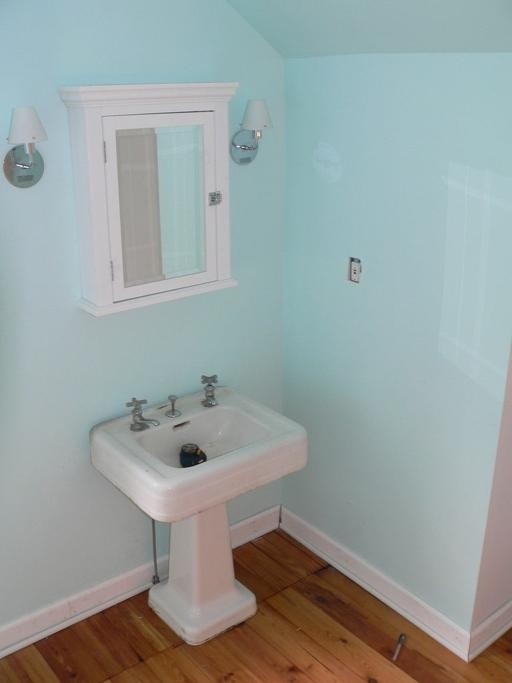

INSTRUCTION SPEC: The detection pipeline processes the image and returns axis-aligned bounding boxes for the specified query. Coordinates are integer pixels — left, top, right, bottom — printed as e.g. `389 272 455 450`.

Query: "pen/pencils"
392 633 406 662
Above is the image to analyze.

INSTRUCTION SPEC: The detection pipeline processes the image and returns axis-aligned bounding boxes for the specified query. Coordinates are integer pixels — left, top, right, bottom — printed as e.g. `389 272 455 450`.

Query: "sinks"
89 386 310 525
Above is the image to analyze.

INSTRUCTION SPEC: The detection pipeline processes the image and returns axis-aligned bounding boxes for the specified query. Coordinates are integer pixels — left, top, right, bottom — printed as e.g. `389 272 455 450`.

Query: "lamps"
229 95 270 166
2 105 49 185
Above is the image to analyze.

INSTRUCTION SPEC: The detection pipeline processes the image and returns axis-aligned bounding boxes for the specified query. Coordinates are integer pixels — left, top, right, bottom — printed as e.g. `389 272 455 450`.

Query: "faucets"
125 397 161 433
199 374 218 408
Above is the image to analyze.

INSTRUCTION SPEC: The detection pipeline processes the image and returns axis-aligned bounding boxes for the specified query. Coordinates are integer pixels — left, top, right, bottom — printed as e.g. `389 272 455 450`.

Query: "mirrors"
99 109 217 304
